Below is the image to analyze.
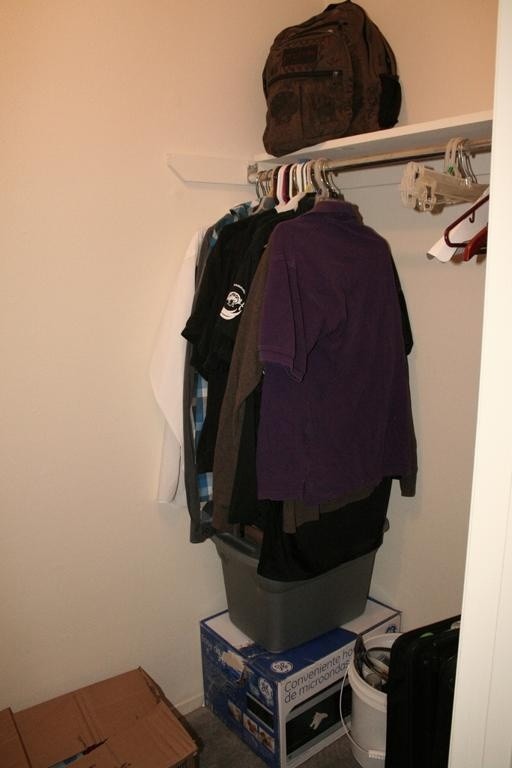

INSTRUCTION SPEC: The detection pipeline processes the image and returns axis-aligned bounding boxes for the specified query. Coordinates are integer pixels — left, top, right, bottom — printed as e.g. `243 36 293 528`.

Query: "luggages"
384 614 461 768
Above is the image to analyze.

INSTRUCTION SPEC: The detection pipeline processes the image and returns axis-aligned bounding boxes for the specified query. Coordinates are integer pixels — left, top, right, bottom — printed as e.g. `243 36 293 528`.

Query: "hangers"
392 153 490 266
241 157 346 214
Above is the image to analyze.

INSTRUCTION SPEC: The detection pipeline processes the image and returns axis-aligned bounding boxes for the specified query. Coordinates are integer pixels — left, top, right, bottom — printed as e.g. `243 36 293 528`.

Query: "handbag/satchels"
262 0 402 157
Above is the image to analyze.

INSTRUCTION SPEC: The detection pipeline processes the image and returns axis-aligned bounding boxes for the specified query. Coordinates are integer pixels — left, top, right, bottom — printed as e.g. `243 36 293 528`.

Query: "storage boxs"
0 666 198 768
199 595 402 767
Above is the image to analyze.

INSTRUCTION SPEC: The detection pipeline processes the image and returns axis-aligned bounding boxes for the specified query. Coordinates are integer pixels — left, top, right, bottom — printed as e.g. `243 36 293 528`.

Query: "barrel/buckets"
337 632 413 766
